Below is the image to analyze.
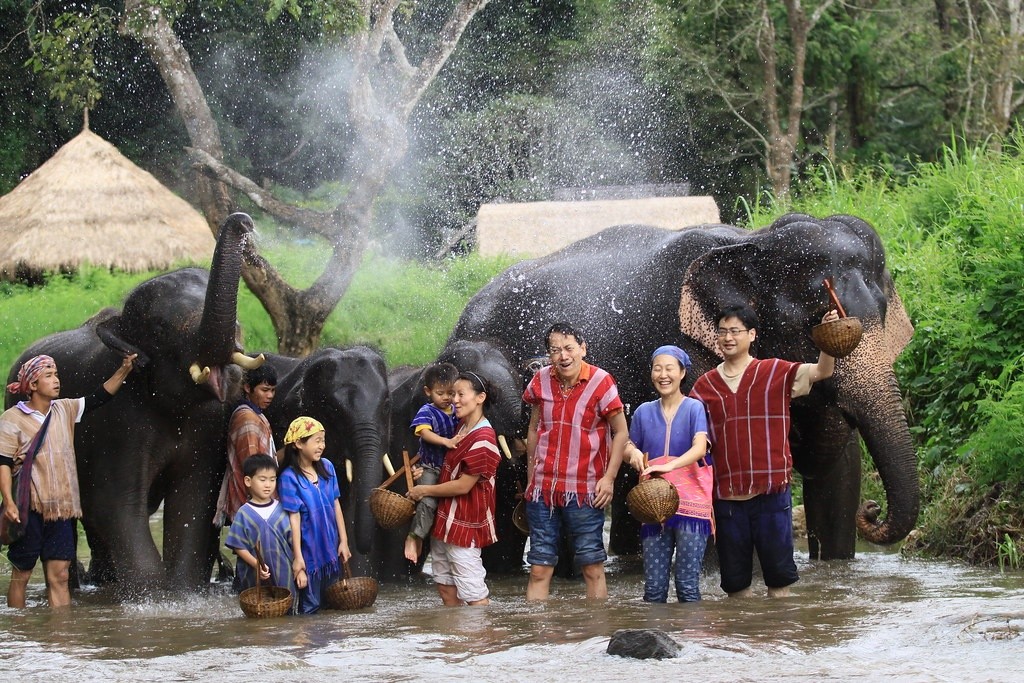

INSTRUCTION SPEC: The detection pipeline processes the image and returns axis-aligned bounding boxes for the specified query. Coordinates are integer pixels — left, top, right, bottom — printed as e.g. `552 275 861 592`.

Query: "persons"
224 453 308 615
621 345 715 602
213 360 279 525
278 417 352 615
688 305 838 596
404 372 501 608
522 322 629 600
403 360 464 568
0 353 138 608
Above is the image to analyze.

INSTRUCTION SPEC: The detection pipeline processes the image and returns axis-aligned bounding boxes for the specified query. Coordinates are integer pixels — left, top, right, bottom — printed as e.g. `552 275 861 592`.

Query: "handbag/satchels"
0 410 53 546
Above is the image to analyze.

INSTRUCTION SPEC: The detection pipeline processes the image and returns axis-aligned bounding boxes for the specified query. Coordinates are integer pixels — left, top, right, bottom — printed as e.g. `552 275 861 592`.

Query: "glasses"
717 328 748 336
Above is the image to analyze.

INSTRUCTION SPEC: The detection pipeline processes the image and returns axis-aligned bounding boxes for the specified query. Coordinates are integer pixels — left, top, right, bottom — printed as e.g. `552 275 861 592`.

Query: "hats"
283 417 324 444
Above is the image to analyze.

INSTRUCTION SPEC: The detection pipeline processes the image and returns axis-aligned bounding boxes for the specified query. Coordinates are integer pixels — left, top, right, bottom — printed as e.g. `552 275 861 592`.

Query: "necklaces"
472 414 483 428
723 368 743 378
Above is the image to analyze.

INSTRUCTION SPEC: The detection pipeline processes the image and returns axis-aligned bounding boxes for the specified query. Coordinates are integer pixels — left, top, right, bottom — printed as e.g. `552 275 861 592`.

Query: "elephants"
387 341 528 573
246 347 392 560
4 212 255 597
454 213 919 562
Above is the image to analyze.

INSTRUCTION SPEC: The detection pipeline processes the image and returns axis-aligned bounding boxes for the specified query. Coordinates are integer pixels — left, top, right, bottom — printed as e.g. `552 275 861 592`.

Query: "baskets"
512 481 531 536
625 451 679 526
369 449 423 530
238 540 292 618
324 554 379 610
810 276 862 359
